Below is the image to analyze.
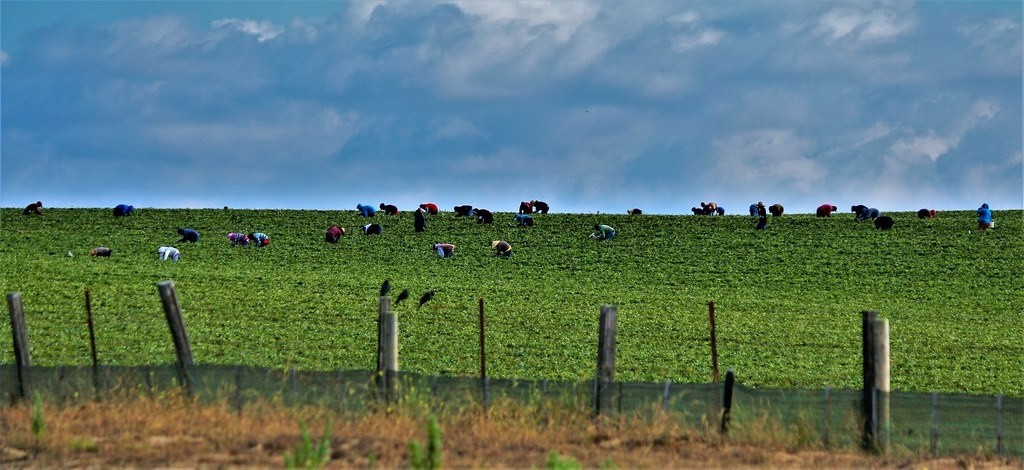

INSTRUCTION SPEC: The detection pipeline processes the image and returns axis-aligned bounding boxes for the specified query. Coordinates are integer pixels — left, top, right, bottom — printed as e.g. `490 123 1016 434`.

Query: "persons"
977 203 992 230
513 200 549 227
492 241 512 260
325 224 345 244
851 205 894 230
227 232 269 248
355 203 376 219
433 243 456 259
23 201 43 216
918 209 936 219
91 247 113 258
158 246 181 262
176 228 199 244
113 204 134 218
380 203 398 216
627 208 643 215
414 203 438 233
454 205 493 224
360 222 383 235
749 201 784 229
590 224 615 240
692 202 725 217
816 204 837 217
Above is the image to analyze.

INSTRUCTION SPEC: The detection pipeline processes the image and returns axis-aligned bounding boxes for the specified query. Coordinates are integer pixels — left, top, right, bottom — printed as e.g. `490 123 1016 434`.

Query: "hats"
492 241 500 249
340 227 346 234
